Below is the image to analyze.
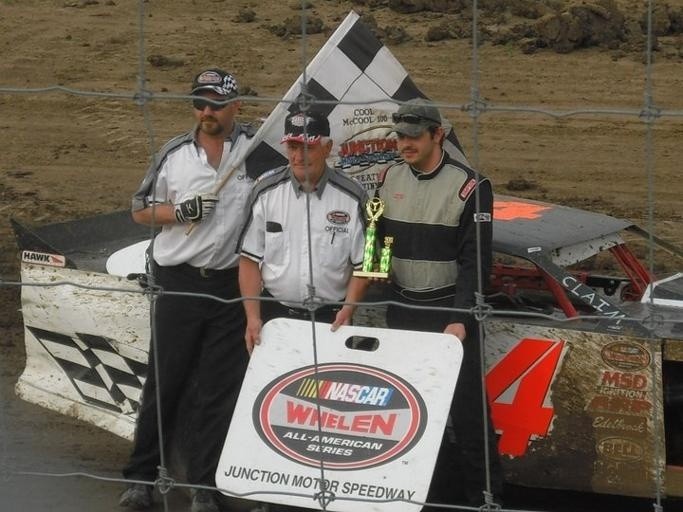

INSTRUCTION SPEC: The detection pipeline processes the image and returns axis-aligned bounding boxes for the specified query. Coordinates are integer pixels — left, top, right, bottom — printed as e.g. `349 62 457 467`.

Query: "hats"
385 98 442 138
190 67 238 99
279 111 329 145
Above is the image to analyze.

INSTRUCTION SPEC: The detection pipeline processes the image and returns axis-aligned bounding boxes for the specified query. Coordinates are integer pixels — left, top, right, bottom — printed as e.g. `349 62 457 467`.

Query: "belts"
263 289 344 318
176 263 238 279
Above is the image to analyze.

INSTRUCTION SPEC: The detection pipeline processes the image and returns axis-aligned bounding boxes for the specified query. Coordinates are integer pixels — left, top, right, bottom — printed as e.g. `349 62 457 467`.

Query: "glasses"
391 110 441 125
192 97 229 110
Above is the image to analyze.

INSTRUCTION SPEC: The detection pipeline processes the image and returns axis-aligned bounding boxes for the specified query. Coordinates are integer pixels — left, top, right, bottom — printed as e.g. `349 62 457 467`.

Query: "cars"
4 183 683 501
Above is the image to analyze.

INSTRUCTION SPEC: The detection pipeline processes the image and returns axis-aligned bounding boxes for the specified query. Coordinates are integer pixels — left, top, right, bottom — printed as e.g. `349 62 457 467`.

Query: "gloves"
173 194 219 224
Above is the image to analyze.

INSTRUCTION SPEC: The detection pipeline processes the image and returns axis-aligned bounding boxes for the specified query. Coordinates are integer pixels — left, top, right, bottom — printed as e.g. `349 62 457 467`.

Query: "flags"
227 12 473 208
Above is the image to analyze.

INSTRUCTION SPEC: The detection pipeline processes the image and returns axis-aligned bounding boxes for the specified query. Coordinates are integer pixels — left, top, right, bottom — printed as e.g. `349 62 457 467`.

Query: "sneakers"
191 490 220 511
120 483 155 510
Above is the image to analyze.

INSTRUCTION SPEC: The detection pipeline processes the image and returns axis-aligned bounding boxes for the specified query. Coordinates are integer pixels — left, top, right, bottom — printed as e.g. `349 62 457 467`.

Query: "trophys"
350 192 394 281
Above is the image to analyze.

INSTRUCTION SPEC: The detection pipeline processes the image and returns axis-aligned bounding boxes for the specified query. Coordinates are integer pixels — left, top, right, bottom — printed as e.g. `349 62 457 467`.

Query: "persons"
232 108 376 512
369 97 507 511
113 67 255 512
